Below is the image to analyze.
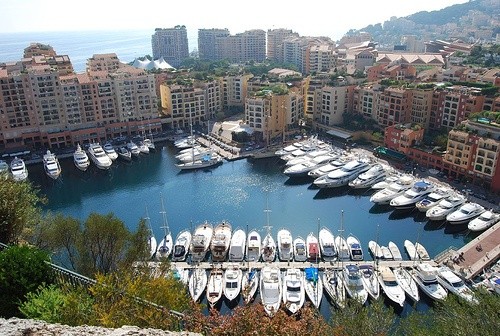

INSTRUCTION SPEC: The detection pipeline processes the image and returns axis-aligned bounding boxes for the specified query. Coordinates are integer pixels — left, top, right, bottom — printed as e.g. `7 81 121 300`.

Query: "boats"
0 161 9 181
87 143 112 171
261 190 430 261
274 134 499 232
282 259 306 314
174 136 222 169
170 219 261 264
104 139 155 162
373 265 406 307
153 266 259 310
472 277 500 315
342 264 368 306
259 261 283 318
41 150 61 180
73 143 90 172
434 267 479 307
403 264 448 303
10 157 28 182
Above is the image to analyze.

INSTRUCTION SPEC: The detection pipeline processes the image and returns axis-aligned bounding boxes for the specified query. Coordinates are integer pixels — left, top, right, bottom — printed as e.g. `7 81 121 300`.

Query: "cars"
245 147 254 150
462 189 474 196
473 193 488 200
255 145 262 149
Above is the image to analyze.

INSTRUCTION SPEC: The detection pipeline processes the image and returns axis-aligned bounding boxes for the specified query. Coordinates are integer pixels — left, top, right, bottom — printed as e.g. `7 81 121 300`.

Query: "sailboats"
393 223 422 303
135 192 173 261
356 224 381 302
323 209 346 309
303 218 323 309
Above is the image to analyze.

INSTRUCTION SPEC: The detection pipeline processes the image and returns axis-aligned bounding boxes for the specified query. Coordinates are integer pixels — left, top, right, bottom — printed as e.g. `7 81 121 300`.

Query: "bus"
324 130 353 145
